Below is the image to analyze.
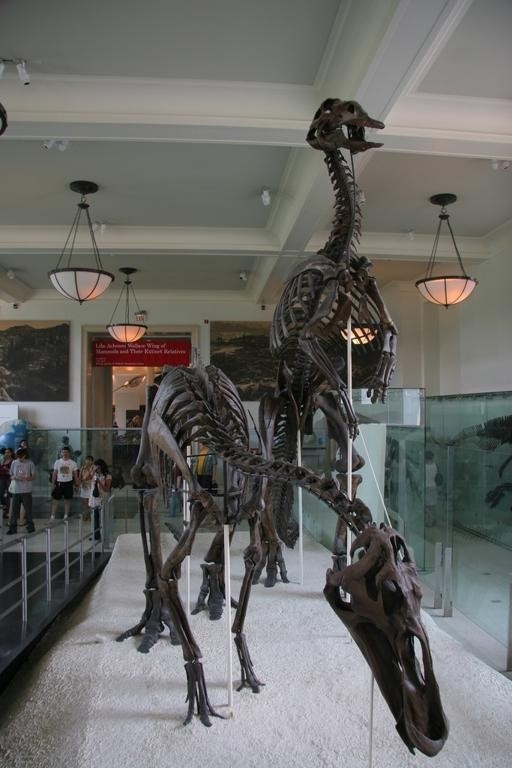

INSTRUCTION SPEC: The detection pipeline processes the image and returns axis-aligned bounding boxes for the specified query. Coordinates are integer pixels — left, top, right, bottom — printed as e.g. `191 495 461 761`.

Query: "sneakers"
7 526 35 536
43 518 56 524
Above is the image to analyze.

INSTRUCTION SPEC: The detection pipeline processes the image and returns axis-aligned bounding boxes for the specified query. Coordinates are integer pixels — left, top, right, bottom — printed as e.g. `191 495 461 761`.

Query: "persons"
1 436 113 542
113 420 120 439
194 439 217 496
384 434 421 513
164 459 184 517
411 448 440 528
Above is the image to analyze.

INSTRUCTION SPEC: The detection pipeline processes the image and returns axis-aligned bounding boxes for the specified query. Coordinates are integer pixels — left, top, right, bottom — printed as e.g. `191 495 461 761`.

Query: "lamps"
47 181 114 305
105 267 148 345
415 193 478 310
